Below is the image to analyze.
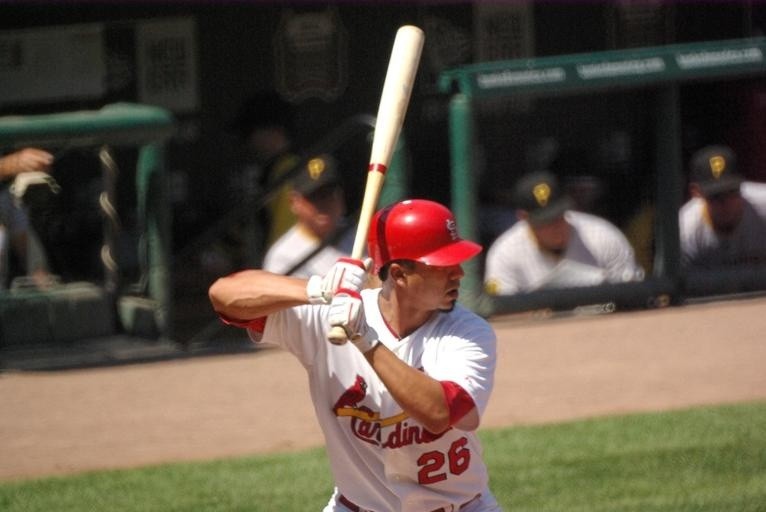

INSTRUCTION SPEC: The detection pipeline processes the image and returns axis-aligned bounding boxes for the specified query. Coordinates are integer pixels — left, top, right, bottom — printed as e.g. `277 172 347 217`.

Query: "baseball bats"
327 23 427 346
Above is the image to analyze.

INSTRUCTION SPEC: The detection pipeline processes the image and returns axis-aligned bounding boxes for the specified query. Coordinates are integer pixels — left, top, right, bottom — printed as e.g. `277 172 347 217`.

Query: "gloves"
306 258 373 304
326 290 380 353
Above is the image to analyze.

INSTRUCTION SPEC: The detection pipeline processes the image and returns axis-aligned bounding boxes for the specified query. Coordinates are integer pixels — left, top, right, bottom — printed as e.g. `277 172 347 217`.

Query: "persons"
0 135 65 288
656 146 766 302
233 88 305 271
479 168 648 314
264 155 365 275
207 201 502 512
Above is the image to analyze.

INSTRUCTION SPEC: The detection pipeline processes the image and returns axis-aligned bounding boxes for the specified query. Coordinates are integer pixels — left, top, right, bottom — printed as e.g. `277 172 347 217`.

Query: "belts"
338 493 483 512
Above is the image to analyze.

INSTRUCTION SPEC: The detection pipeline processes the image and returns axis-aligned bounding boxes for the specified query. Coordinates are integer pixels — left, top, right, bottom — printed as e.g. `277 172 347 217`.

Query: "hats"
292 154 343 196
513 170 578 224
688 148 741 198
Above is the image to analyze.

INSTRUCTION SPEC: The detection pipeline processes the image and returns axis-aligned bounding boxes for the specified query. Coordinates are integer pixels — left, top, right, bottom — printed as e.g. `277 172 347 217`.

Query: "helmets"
367 200 485 276
